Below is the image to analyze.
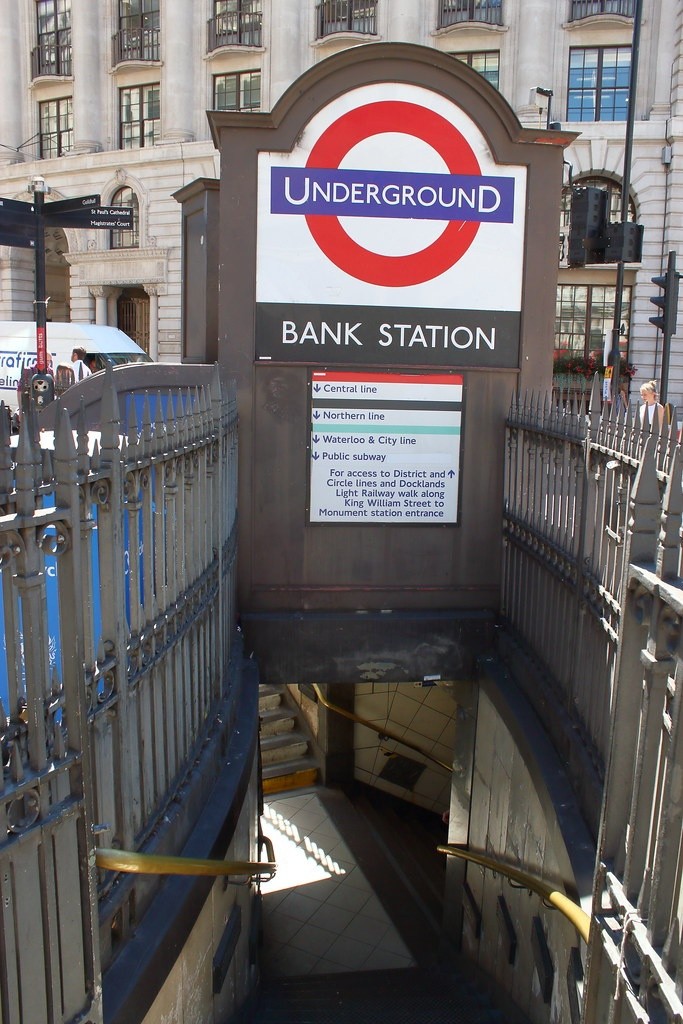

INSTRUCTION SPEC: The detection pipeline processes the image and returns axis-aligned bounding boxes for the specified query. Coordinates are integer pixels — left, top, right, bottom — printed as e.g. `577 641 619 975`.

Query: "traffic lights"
648 272 680 334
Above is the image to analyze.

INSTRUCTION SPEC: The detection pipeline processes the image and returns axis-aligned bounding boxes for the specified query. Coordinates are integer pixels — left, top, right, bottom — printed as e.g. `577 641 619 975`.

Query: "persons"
84 354 103 374
614 379 628 417
632 378 666 431
15 350 55 426
67 348 93 386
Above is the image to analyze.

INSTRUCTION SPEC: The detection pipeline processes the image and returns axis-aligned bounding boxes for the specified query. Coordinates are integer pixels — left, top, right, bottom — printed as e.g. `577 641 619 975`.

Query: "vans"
0 320 155 425
